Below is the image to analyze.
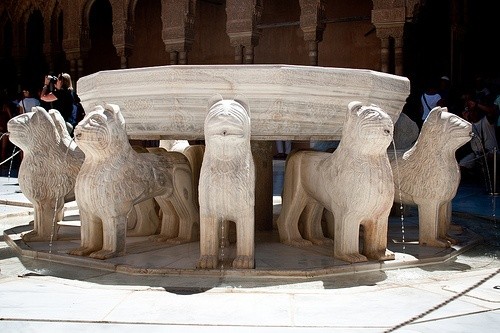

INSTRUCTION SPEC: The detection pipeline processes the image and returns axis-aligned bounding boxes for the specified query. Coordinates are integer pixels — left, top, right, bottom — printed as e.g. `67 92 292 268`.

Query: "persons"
2 71 79 136
124 66 500 236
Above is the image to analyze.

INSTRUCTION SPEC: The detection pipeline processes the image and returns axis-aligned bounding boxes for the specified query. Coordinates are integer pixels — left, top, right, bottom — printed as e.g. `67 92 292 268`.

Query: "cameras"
50 76 58 84
